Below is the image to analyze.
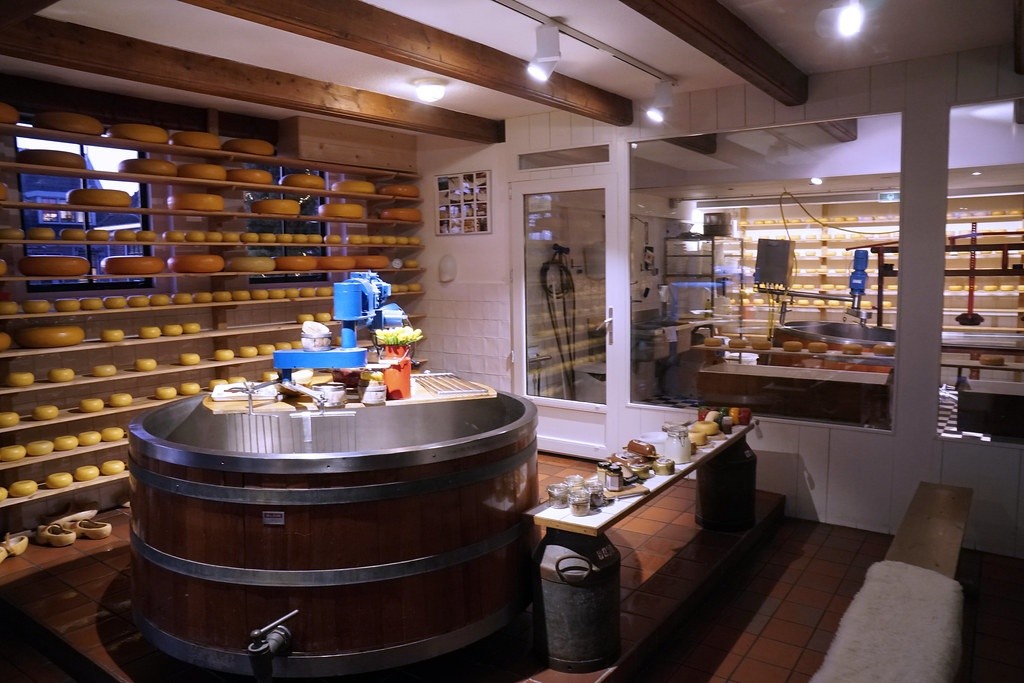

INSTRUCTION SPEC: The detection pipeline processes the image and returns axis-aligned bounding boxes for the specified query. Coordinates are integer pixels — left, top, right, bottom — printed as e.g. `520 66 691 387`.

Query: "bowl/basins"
292 369 313 384
302 320 329 334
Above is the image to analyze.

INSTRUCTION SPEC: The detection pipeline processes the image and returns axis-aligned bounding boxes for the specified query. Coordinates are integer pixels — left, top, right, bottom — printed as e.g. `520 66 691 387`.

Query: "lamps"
414 78 450 103
815 0 862 36
646 80 673 122
526 24 562 82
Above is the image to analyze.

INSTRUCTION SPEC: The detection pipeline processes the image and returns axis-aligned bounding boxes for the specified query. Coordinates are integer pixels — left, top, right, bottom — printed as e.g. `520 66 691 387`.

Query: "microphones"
849 250 868 309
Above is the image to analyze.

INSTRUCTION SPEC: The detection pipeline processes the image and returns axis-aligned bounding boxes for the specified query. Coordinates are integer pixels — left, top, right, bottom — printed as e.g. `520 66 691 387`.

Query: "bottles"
665 426 691 464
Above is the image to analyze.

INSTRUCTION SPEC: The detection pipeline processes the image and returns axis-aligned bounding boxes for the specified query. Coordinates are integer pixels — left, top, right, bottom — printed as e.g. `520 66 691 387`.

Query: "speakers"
755 239 796 285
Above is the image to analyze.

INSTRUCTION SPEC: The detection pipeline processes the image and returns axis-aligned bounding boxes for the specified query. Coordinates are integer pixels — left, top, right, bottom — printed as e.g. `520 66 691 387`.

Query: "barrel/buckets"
773 319 897 351
125 389 538 678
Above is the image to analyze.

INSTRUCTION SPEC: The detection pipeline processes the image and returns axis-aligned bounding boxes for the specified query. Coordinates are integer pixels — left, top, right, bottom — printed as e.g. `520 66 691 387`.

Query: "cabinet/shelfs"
663 236 714 318
0 122 426 587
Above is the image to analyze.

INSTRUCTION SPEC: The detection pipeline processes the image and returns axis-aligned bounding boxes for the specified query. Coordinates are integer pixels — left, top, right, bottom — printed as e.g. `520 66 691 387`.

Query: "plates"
211 383 276 400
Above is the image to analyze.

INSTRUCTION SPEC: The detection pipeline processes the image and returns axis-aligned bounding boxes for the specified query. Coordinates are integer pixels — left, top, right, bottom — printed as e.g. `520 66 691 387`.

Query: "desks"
693 341 1024 385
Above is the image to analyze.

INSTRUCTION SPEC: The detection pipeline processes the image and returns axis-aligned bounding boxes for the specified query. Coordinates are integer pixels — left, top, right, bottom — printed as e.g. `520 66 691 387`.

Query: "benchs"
809 481 974 683
